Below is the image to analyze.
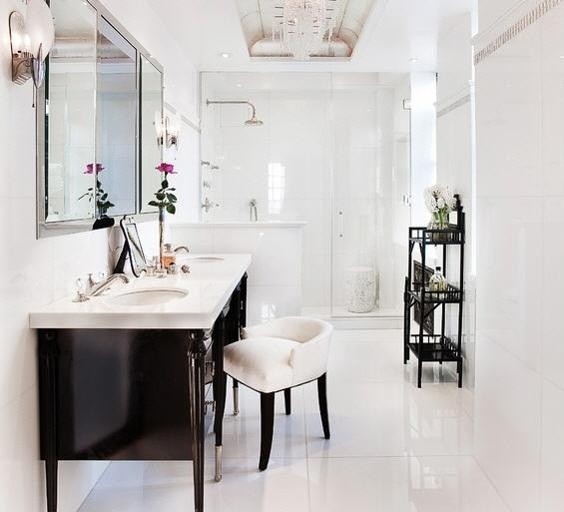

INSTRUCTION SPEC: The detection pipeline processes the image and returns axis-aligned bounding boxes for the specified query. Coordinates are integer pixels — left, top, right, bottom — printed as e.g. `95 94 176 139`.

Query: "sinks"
94 285 190 305
179 256 224 262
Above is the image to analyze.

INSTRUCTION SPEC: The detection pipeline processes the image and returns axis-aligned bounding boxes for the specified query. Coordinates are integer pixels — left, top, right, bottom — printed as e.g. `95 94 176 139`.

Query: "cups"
145 255 158 277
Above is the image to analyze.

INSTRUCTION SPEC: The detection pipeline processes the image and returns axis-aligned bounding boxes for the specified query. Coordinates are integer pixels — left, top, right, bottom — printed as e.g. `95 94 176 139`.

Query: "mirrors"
113 215 148 277
35 0 165 241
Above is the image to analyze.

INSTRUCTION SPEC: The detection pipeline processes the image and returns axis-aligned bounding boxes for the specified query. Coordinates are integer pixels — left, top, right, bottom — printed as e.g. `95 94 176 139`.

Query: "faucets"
88 273 129 297
174 246 189 253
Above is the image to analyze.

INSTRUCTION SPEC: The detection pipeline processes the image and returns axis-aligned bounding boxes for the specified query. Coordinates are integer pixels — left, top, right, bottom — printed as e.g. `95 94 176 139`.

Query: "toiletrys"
162 242 177 269
429 267 447 297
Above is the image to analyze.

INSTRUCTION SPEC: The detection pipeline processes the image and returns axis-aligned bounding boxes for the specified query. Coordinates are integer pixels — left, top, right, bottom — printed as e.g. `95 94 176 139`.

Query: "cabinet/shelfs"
403 193 465 388
29 252 253 512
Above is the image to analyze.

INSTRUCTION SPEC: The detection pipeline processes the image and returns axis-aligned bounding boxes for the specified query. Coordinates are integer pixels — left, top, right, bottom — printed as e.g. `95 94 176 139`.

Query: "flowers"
423 184 457 213
78 163 115 214
148 163 177 215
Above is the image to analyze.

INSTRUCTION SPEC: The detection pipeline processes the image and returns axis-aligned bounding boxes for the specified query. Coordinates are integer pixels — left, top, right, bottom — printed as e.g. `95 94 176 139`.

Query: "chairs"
213 317 333 471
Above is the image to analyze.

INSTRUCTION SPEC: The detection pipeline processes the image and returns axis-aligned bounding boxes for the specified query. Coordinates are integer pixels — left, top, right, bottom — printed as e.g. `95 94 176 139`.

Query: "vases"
427 207 451 241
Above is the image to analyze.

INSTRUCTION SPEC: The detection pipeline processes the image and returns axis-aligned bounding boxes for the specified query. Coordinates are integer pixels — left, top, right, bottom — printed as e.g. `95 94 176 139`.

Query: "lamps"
235 0 375 62
9 0 55 89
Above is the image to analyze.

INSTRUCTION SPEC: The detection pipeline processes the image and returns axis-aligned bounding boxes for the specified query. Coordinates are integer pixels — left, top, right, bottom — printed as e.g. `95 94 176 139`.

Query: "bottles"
429 266 449 300
